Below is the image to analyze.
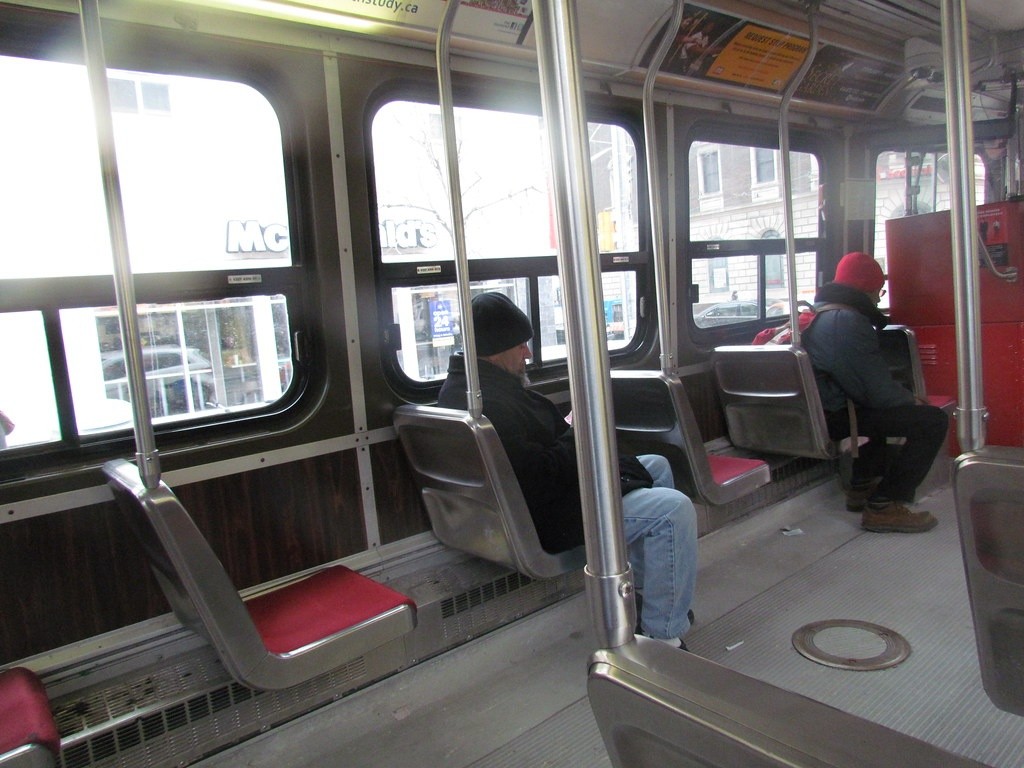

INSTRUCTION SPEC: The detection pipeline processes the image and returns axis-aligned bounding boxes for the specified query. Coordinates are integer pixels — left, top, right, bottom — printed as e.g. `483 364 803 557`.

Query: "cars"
100 343 215 418
692 296 787 328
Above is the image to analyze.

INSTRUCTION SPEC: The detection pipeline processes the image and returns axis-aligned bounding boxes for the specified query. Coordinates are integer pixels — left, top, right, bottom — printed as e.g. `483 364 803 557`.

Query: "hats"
834 252 890 293
471 291 533 357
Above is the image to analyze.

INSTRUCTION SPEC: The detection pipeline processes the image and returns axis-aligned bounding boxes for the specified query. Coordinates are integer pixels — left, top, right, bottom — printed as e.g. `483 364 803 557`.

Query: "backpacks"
752 301 861 346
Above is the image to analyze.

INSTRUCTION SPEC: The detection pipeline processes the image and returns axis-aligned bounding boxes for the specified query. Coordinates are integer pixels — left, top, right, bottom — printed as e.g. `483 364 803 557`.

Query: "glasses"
876 289 886 298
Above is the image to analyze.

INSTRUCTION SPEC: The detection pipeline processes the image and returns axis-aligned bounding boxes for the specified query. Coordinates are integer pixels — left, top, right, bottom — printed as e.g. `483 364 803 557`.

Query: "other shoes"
635 603 694 636
845 477 880 513
637 624 688 652
862 500 938 534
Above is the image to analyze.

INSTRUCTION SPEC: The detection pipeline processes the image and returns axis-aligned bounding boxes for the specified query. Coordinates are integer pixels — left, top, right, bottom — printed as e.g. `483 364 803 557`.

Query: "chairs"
394 403 585 579
952 445 1024 717
100 457 416 691
585 634 993 768
607 369 771 508
872 325 956 414
0 666 62 768
714 344 868 459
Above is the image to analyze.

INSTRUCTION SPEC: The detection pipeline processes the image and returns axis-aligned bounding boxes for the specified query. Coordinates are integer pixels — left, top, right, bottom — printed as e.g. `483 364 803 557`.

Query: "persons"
732 290 738 300
802 251 949 532
438 292 695 654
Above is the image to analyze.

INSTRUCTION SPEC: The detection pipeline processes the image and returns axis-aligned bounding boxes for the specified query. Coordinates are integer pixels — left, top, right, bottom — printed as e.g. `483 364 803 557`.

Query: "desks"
225 362 256 404
